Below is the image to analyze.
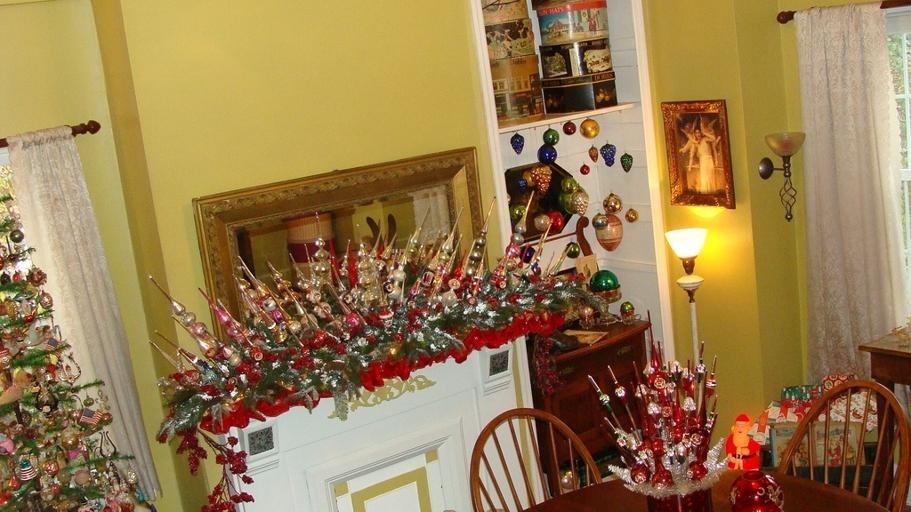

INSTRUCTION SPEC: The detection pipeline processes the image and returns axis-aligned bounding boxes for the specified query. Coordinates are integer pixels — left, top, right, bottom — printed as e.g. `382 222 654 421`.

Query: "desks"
858 325 911 508
516 467 891 512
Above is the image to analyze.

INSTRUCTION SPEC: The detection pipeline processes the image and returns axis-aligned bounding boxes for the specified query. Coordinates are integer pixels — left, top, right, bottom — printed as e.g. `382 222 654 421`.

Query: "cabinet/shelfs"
526 314 652 472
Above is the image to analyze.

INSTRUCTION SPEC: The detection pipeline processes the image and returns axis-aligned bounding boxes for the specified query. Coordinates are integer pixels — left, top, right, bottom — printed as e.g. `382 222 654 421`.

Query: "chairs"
778 379 911 512
470 407 602 512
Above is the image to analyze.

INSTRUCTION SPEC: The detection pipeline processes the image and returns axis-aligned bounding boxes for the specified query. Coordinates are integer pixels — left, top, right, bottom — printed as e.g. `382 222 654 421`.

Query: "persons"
724 414 762 473
678 115 721 195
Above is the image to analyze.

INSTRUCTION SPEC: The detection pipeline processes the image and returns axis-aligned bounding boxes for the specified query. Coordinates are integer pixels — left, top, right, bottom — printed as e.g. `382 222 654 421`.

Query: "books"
769 419 867 469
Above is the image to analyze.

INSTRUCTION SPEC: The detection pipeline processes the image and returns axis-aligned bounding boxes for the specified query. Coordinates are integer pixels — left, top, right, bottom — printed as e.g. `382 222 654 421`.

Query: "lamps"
664 228 705 380
758 132 805 222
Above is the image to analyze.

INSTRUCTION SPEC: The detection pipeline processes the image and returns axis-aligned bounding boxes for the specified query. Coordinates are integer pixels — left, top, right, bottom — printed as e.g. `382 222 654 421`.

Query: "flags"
1 154 145 512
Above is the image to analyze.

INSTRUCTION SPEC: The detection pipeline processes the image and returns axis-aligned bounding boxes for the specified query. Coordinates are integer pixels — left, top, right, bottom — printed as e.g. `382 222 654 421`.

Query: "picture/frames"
191 145 490 349
661 98 736 209
